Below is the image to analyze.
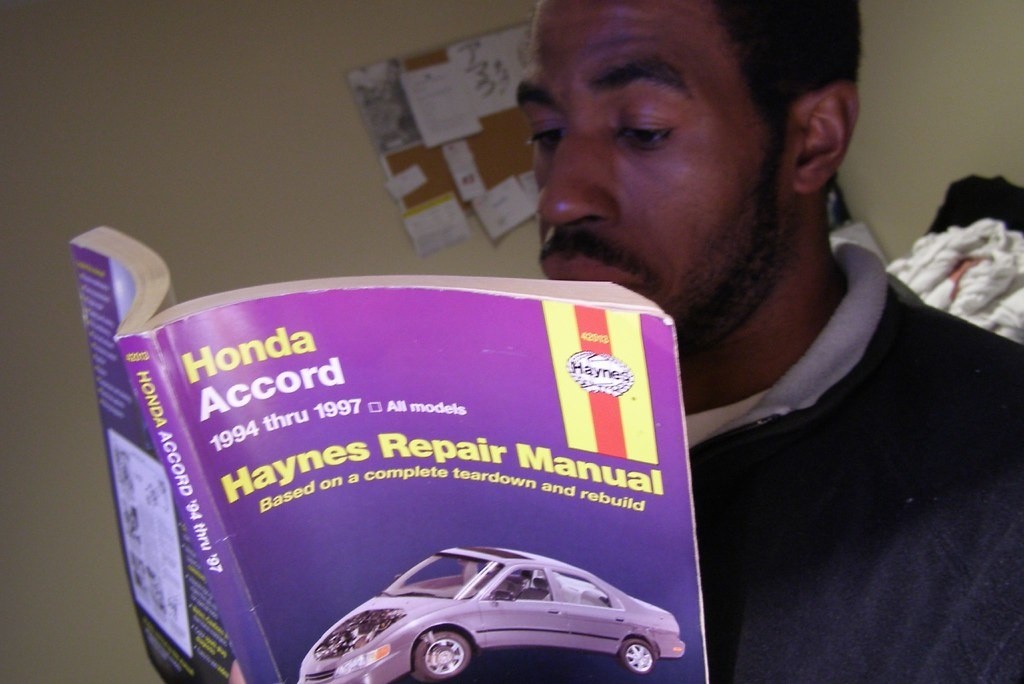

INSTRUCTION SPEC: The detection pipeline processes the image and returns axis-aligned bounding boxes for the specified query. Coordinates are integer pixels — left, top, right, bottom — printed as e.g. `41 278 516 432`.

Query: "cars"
297 548 685 683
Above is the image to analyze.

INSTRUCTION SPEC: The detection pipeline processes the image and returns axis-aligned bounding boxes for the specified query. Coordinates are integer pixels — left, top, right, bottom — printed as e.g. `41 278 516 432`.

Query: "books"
66 225 709 684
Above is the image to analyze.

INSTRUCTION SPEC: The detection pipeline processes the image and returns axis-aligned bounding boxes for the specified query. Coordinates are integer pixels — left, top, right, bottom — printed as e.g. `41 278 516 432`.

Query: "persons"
512 0 1024 684
